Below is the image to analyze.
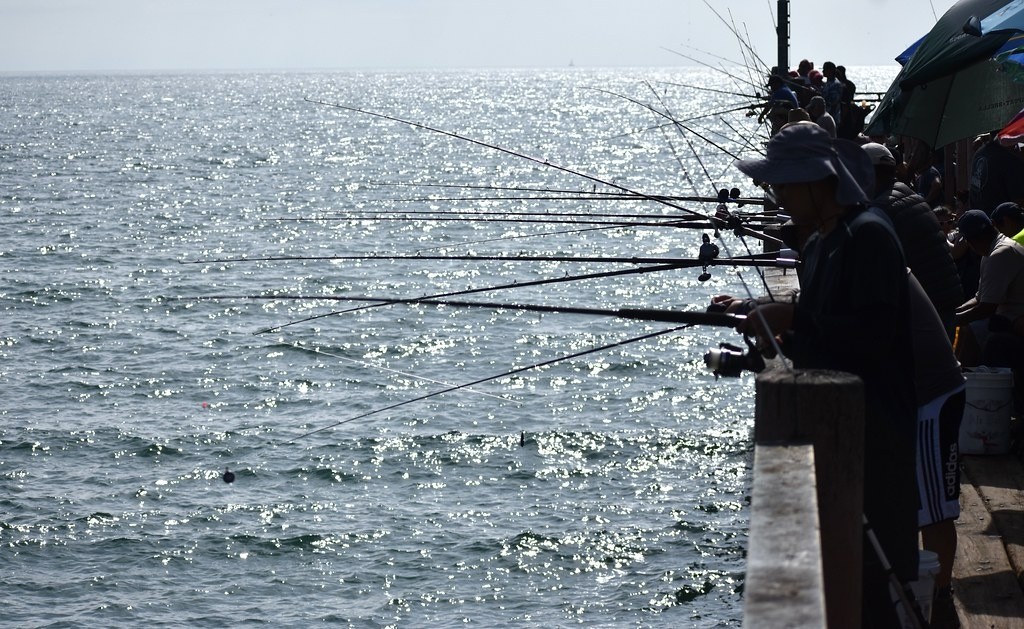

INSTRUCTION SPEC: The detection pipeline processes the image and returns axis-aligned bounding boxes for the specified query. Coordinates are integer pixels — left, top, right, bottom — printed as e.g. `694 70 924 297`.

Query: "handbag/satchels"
961 366 1014 455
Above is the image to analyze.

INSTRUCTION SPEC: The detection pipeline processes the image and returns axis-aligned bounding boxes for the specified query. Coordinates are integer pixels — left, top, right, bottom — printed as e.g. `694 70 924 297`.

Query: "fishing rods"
265 1 801 440
656 96 931 627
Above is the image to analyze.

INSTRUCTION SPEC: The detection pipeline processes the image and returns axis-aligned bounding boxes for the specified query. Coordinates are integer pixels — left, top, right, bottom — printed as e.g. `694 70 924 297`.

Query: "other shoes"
929 584 961 629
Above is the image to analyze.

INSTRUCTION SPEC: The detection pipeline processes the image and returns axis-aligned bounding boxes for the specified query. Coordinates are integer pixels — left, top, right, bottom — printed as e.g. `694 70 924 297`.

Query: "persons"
698 61 1023 353
735 119 967 629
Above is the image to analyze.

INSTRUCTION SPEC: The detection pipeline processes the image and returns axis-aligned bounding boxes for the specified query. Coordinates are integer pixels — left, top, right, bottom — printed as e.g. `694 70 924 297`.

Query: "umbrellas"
862 0 1024 154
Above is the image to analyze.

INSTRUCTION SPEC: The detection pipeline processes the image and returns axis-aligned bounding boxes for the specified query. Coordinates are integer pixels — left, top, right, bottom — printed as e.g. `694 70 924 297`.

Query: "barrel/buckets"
959 368 1014 455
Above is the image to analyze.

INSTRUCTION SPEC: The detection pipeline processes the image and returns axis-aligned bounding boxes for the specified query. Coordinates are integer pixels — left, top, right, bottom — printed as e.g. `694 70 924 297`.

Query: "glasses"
939 221 954 226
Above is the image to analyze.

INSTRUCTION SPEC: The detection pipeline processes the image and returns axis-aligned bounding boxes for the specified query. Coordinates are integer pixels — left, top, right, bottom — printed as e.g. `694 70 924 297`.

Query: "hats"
797 60 813 75
805 96 826 110
832 138 877 193
958 210 993 238
837 66 845 76
860 141 896 167
738 125 870 207
809 72 823 80
764 75 784 87
789 72 798 77
780 107 812 130
